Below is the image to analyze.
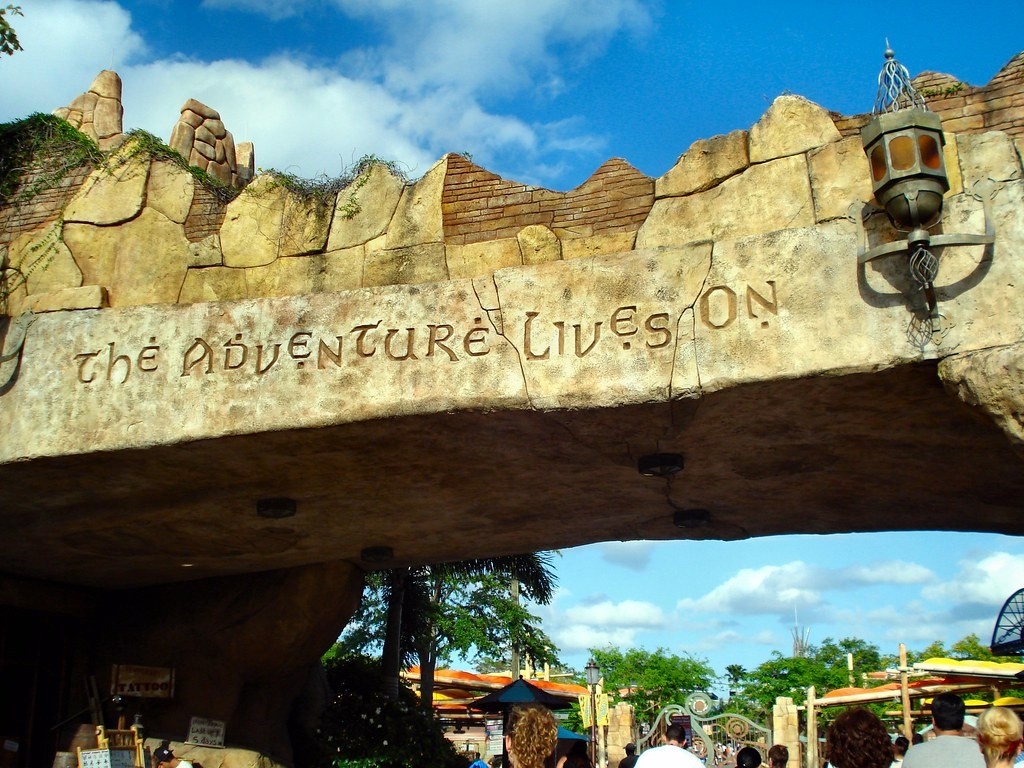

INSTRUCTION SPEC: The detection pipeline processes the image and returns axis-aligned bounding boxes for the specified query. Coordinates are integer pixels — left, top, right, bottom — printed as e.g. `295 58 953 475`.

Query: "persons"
735 747 762 768
714 742 741 765
976 707 1024 768
634 724 706 768
618 743 640 768
153 747 193 768
824 707 896 768
890 737 909 768
768 745 789 768
473 753 481 762
902 692 987 768
912 734 924 746
469 754 502 768
505 702 591 768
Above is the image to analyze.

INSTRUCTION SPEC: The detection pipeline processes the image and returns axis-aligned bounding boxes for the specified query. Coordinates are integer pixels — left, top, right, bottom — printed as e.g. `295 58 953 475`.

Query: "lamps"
637 452 683 477
855 35 999 352
361 547 393 561
257 497 296 518
674 508 711 529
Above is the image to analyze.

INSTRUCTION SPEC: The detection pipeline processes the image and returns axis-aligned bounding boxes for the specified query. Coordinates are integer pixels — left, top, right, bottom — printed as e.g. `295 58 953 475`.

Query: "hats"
153 747 174 762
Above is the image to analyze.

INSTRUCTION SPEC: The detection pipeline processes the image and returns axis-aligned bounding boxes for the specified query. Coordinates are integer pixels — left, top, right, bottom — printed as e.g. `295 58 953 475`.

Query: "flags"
578 694 592 728
595 693 610 726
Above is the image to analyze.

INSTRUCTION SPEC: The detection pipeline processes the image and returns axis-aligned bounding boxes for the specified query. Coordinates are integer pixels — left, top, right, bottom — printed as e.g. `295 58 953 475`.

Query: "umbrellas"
469 674 573 710
557 725 589 742
